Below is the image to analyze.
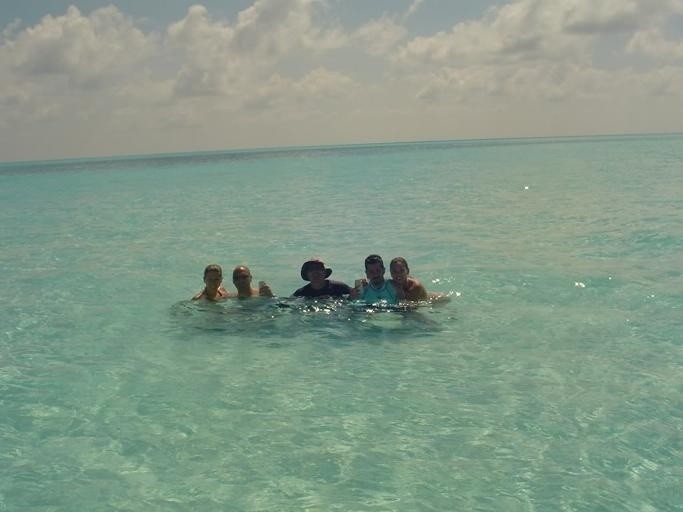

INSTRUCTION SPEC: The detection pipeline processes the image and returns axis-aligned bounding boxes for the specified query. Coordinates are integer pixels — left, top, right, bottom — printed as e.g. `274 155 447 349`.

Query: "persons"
190 264 232 304
349 254 406 304
358 256 429 307
230 265 276 298
289 258 354 299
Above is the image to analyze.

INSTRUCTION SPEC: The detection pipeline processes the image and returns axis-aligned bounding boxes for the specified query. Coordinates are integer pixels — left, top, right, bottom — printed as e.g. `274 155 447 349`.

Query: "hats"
301 261 333 280
